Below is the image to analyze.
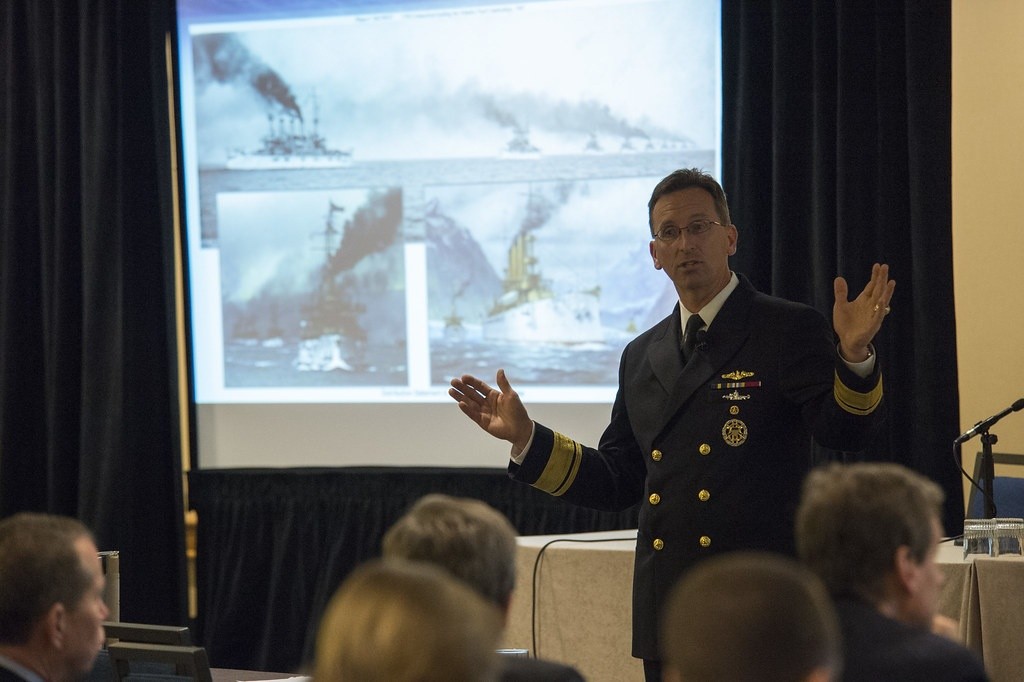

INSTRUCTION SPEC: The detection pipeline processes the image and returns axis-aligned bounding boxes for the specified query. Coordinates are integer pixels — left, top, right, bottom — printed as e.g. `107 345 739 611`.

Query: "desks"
498 529 1024 682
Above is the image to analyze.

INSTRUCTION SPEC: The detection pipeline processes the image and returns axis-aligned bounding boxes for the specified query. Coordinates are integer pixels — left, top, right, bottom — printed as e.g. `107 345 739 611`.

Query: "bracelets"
866 346 872 359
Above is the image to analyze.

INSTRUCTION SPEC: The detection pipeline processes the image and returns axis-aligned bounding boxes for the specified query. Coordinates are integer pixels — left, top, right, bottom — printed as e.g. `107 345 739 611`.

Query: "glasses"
653 219 729 241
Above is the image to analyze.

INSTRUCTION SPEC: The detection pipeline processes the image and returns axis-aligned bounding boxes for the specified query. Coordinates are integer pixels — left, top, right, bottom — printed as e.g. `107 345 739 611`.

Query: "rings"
873 304 879 311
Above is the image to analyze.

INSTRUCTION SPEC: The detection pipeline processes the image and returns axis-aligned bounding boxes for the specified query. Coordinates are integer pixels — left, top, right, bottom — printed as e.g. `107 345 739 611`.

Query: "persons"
448 168 896 682
313 559 500 682
796 462 992 682
0 514 111 682
659 557 845 682
383 492 588 682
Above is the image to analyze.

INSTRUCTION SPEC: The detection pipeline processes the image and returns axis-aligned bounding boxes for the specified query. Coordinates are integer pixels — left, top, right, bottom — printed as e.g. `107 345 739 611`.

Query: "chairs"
964 452 1023 555
91 623 213 682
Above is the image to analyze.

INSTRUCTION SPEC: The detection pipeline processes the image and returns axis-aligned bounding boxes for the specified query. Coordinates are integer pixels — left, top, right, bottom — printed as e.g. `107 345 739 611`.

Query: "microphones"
696 330 709 350
955 398 1024 445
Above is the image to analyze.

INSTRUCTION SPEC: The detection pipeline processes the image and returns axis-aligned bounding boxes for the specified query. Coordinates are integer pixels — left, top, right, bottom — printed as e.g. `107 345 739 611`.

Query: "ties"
681 314 707 366
